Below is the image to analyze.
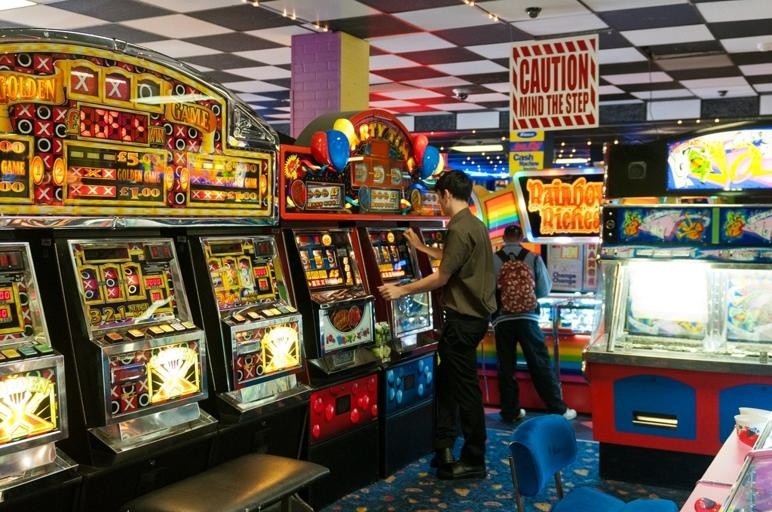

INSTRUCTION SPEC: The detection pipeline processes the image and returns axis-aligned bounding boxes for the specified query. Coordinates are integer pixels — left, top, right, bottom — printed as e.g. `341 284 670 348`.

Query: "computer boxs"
528 9 539 18
719 92 726 96
456 93 467 100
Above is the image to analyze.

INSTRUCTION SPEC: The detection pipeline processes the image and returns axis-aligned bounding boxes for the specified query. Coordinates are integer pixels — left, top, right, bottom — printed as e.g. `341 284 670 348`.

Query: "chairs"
563 407 578 419
428 446 456 468
506 408 527 423
435 458 487 481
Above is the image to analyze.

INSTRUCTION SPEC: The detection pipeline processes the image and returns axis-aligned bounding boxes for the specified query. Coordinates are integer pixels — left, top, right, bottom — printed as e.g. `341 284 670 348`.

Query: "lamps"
508 413 677 511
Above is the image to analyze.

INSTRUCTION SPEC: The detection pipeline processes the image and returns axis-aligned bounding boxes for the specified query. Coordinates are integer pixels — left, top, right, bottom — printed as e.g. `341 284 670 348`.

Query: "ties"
536 240 772 358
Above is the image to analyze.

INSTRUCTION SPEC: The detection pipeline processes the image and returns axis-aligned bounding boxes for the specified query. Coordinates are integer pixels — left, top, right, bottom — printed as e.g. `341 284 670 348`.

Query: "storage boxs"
122 453 331 511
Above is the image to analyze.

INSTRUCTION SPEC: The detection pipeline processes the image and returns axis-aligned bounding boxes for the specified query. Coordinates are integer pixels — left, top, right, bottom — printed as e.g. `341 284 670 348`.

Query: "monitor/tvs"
536 240 772 358
370 231 416 282
200 236 279 312
2 241 54 345
67 238 194 339
293 232 357 291
423 231 446 274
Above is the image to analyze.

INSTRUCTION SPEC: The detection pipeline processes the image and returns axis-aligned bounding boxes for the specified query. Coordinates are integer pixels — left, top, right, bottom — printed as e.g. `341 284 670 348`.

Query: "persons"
490 225 577 423
377 170 498 479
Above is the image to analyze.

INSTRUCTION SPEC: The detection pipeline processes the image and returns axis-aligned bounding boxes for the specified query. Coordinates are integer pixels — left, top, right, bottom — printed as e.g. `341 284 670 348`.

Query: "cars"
734 405 772 450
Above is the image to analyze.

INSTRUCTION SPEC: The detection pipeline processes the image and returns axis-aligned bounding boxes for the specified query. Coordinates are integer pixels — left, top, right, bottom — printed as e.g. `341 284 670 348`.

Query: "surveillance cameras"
719 92 726 96
528 9 539 18
456 93 467 100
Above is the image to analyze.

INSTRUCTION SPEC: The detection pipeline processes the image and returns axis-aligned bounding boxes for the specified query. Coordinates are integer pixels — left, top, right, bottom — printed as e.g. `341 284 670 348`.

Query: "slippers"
495 248 538 314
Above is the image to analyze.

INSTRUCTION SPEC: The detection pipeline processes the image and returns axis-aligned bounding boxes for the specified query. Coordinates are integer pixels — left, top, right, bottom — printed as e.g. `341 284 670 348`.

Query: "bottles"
503 226 523 235
